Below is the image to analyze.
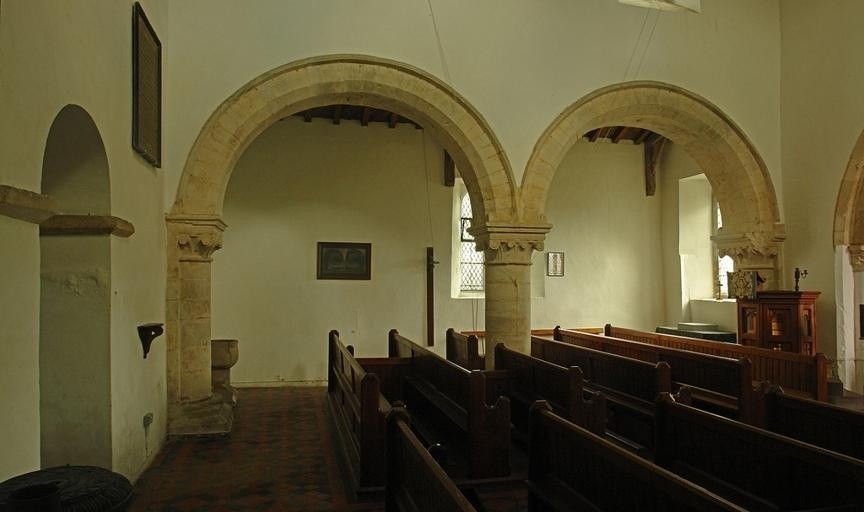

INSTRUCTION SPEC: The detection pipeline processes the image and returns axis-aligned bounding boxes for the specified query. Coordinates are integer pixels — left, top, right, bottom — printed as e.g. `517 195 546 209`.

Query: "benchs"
527 400 750 512
328 330 423 504
382 407 481 512
446 327 485 369
766 385 864 461
388 328 511 476
495 340 605 437
553 326 754 398
605 325 827 402
531 334 671 403
656 391 864 512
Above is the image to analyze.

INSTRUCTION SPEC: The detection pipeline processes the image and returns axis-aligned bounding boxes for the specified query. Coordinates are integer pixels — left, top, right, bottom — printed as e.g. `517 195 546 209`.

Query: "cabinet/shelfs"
757 288 822 354
737 296 758 348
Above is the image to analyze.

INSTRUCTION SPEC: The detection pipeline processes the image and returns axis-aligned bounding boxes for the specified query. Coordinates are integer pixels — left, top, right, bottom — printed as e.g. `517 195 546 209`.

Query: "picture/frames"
130 2 163 168
316 240 372 281
546 251 564 277
